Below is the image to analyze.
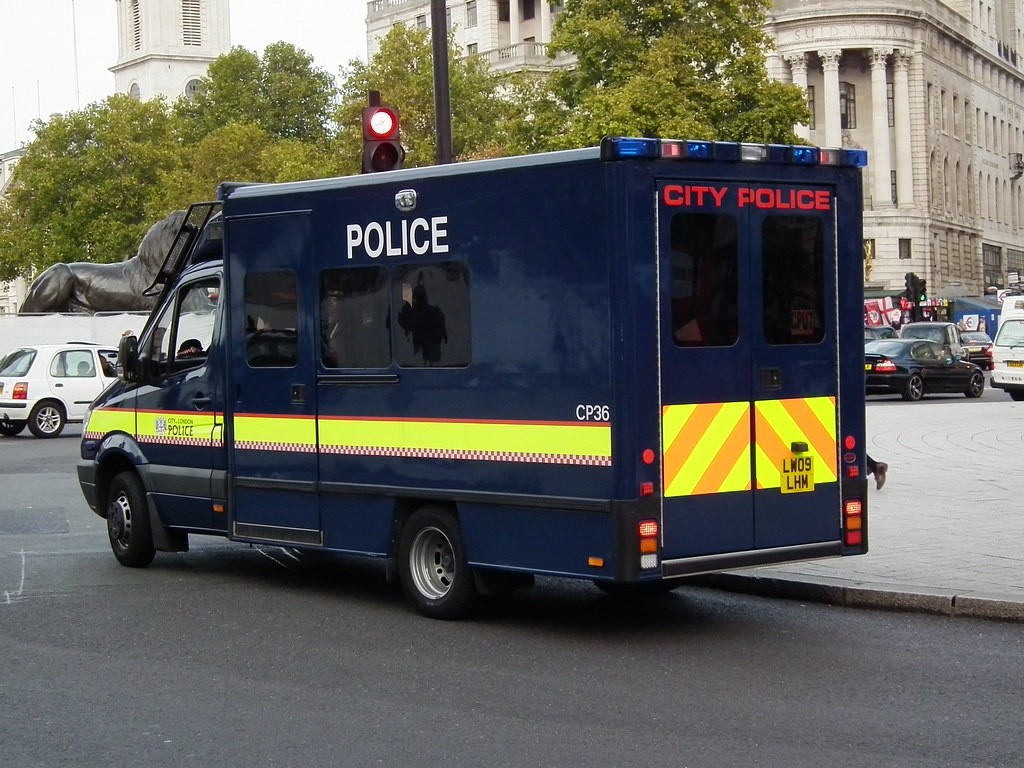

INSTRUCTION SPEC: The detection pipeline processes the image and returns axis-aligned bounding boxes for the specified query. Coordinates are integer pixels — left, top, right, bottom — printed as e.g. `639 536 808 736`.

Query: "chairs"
56 362 71 377
77 361 91 377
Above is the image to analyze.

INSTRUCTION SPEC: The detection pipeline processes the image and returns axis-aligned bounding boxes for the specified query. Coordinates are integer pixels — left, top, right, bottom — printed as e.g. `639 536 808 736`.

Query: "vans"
79 134 868 619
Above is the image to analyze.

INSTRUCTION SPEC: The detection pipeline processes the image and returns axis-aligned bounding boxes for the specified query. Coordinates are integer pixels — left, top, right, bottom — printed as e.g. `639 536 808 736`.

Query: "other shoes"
873 462 887 490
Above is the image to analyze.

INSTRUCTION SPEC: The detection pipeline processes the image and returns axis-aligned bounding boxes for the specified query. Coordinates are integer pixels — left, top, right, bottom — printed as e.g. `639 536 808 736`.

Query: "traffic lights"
360 89 407 174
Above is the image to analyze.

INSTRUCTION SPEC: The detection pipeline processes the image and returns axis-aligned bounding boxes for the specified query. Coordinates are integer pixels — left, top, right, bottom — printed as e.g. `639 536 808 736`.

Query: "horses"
17 209 215 316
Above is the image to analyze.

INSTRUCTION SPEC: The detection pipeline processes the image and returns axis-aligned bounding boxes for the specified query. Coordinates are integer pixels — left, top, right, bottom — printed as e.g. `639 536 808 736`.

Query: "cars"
3 341 123 435
870 299 1024 398
